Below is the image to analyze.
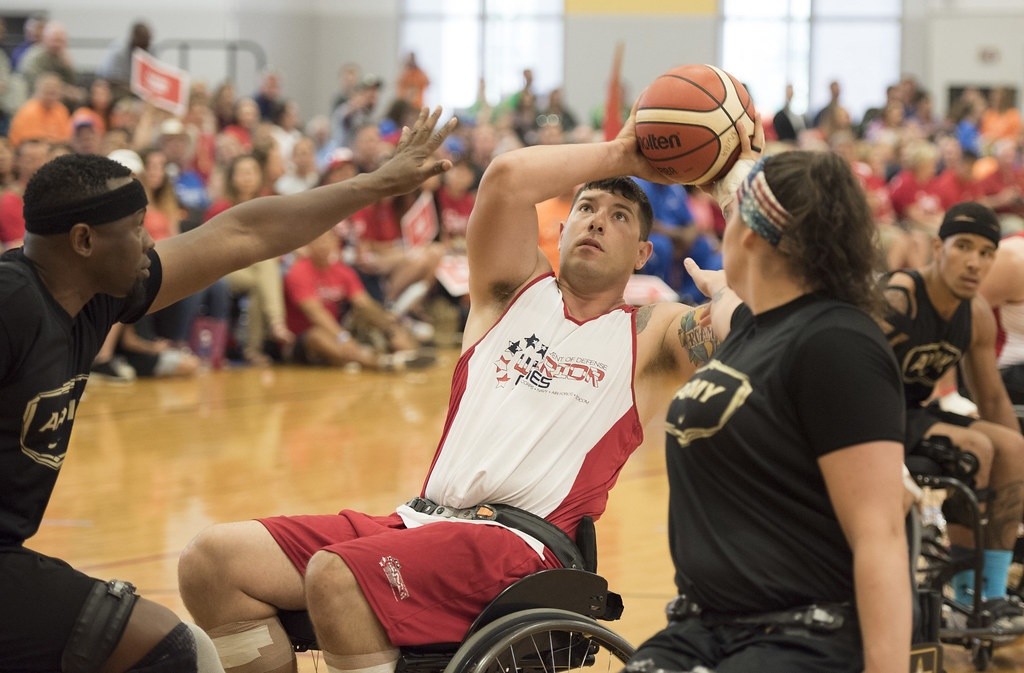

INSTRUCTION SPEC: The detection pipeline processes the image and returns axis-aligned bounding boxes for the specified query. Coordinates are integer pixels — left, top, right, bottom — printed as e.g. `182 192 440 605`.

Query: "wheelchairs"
278 503 638 673
906 431 1024 673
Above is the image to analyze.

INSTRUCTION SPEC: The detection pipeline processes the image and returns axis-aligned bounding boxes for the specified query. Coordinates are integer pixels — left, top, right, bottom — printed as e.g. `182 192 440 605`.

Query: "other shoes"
947 599 1023 641
378 351 435 371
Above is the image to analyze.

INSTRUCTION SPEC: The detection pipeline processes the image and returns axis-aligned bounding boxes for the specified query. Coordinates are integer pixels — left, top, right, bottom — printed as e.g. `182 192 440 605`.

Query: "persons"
0 88 1024 672
0 0 1024 374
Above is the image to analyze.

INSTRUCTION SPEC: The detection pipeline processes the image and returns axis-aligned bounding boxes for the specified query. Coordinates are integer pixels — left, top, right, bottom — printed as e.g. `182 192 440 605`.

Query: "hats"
105 150 144 175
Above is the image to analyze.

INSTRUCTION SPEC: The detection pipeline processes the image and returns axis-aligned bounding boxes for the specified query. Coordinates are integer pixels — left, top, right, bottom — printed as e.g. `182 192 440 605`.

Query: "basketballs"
636 63 756 187
